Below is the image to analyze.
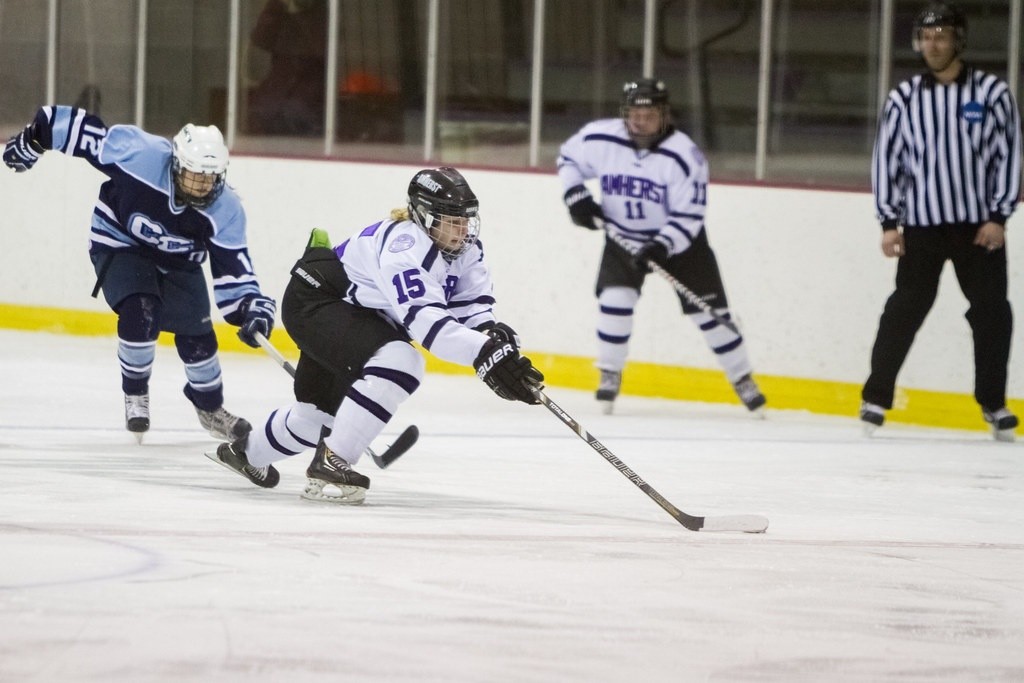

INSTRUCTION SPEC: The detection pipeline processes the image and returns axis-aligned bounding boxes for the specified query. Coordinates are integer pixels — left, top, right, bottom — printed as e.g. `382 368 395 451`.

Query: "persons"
555 78 777 422
204 167 547 507
859 2 1023 441
2 104 277 443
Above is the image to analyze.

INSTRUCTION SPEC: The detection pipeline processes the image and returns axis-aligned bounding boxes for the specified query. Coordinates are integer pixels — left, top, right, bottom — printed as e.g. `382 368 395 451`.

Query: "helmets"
172 123 229 174
912 0 966 54
621 78 669 124
408 167 479 237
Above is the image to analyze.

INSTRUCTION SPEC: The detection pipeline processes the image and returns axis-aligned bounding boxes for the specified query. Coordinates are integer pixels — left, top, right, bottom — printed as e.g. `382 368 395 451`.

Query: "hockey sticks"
253 329 420 469
593 216 744 337
523 377 769 533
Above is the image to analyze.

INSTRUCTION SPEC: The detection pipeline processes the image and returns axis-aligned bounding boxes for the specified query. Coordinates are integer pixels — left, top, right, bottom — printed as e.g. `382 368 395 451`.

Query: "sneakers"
859 400 885 437
123 392 150 446
300 424 370 506
982 408 1018 443
594 368 622 412
734 374 766 419
205 434 280 489
193 403 253 443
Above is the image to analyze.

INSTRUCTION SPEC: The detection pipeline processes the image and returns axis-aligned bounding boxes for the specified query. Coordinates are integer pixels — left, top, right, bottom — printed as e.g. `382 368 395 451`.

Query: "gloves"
3 123 47 174
627 240 667 276
563 184 604 231
473 323 545 407
237 295 277 348
